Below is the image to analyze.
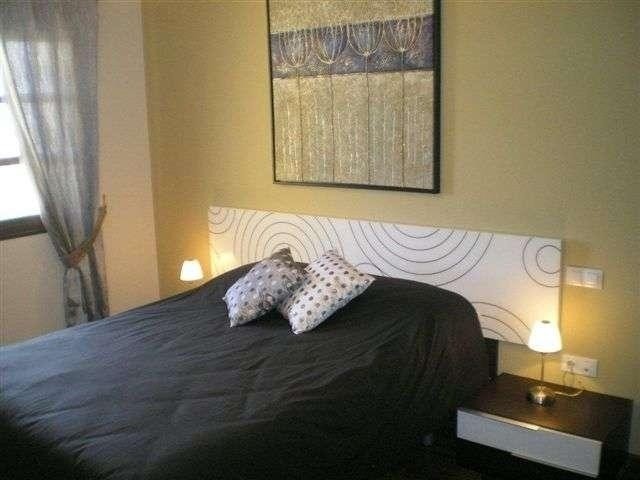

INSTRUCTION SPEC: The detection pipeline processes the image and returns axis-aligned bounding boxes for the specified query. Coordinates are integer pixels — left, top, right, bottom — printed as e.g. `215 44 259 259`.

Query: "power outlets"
562 353 598 378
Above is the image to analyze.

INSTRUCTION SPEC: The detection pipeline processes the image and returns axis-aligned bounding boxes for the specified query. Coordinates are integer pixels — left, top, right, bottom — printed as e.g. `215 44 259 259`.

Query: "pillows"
227 249 309 328
278 249 376 334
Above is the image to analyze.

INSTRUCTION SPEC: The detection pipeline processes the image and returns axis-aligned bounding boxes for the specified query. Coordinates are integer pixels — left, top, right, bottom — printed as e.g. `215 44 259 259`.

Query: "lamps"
526 320 562 407
179 258 203 285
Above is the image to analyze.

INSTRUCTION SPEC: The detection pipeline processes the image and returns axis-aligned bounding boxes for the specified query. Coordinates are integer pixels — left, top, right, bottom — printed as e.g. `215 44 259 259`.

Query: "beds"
0 207 564 480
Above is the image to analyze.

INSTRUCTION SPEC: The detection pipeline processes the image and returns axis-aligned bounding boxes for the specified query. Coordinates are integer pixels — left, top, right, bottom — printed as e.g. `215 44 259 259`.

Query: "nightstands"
452 373 635 480
